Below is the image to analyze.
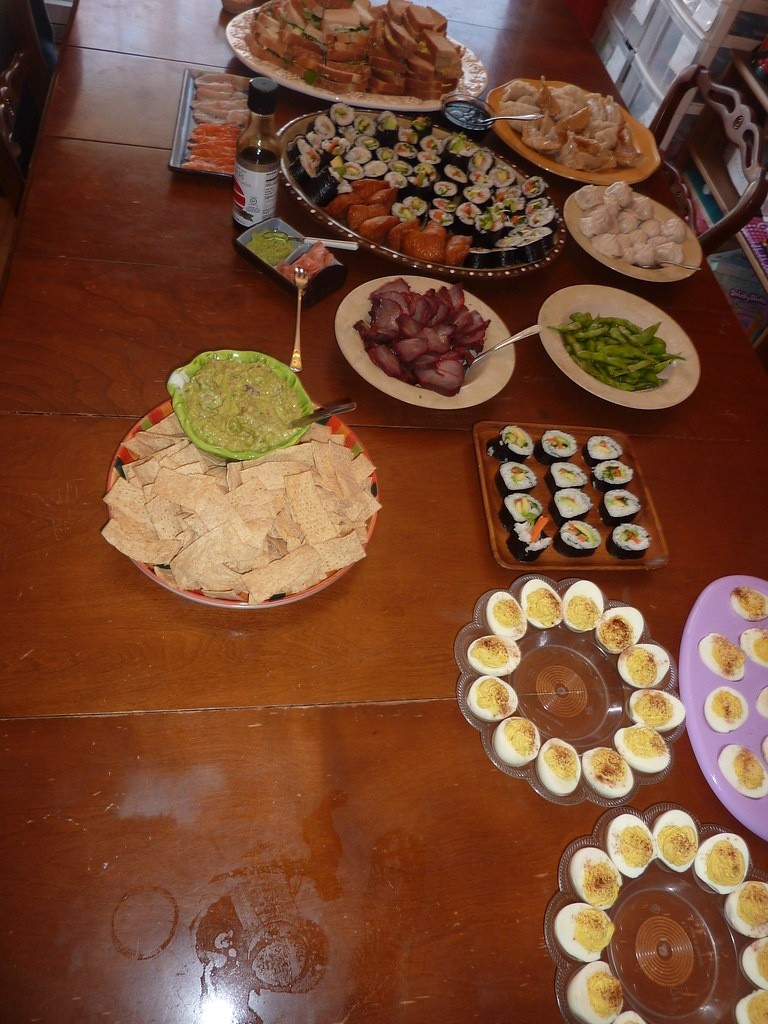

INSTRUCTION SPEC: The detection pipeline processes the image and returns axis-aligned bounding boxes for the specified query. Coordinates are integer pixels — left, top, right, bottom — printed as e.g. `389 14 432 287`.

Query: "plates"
166 65 252 179
484 77 662 186
538 284 702 410
678 573 768 843
453 575 686 809
564 186 703 282
275 108 568 279
543 804 768 1024
106 399 379 609
334 274 515 410
472 420 671 571
224 5 488 112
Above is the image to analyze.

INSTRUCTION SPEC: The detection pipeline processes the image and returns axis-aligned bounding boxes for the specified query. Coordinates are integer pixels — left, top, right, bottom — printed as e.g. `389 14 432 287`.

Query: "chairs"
649 63 768 252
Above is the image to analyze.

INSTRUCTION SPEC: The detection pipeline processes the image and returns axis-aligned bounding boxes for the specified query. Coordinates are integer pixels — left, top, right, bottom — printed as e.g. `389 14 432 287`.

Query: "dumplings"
499 73 646 174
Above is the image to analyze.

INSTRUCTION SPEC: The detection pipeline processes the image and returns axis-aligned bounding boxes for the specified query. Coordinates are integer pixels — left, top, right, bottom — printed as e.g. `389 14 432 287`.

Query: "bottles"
232 76 282 230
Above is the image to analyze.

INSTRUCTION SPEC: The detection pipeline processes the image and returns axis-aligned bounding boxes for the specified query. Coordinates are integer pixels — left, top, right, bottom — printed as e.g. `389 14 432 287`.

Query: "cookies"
100 412 383 608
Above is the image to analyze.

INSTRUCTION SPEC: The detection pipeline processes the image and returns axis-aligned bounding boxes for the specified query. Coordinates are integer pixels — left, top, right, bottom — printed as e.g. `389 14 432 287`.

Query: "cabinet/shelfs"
588 0 768 142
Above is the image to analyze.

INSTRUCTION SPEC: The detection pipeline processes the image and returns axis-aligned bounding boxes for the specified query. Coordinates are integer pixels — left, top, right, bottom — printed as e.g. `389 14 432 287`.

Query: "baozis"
574 178 686 268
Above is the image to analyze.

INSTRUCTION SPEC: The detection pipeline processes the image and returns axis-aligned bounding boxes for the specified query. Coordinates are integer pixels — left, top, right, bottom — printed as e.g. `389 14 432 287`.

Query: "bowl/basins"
234 216 347 304
166 349 315 462
440 95 495 142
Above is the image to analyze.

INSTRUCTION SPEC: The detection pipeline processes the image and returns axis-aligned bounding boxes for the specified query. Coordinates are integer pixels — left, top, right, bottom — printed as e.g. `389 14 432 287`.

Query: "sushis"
486 424 652 563
287 101 557 269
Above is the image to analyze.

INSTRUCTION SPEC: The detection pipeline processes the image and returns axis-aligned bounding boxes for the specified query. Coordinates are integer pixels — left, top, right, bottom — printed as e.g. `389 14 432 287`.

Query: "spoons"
263 230 359 252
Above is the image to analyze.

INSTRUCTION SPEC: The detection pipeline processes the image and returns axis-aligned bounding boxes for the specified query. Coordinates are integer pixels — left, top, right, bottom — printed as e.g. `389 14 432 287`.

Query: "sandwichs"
244 0 461 102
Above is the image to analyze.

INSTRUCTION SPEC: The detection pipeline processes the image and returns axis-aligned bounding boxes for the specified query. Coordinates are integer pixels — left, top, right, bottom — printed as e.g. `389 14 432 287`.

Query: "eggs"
467 578 686 799
699 586 768 799
554 809 768 1024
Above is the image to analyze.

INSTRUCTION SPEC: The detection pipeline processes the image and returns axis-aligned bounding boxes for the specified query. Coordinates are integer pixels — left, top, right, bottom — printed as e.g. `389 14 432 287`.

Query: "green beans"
549 313 689 392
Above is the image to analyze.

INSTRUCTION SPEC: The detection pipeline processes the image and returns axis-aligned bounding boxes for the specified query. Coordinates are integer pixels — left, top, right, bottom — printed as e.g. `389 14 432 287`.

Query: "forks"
289 267 312 373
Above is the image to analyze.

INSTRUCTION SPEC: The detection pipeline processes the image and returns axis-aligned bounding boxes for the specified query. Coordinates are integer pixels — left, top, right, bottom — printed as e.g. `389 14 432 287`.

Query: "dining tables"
0 0 768 1024
